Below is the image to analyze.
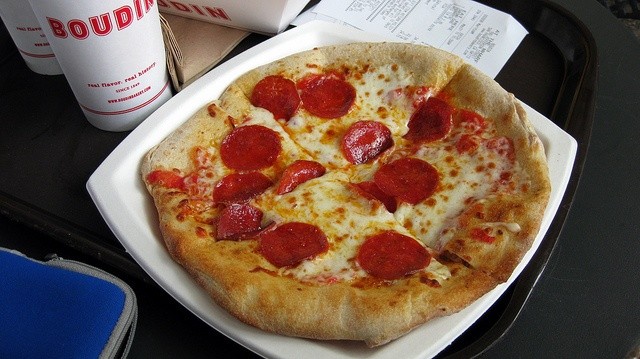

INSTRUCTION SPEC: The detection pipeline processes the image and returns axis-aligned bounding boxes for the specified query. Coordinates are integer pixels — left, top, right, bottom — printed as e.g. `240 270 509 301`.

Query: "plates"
82 20 579 358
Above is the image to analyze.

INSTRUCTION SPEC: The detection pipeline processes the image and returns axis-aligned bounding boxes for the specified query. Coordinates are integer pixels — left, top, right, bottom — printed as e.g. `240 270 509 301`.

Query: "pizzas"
141 41 550 348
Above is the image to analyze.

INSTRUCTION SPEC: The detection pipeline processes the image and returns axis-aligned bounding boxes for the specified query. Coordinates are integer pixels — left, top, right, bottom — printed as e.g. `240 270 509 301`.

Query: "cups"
0 0 64 76
27 0 173 132
